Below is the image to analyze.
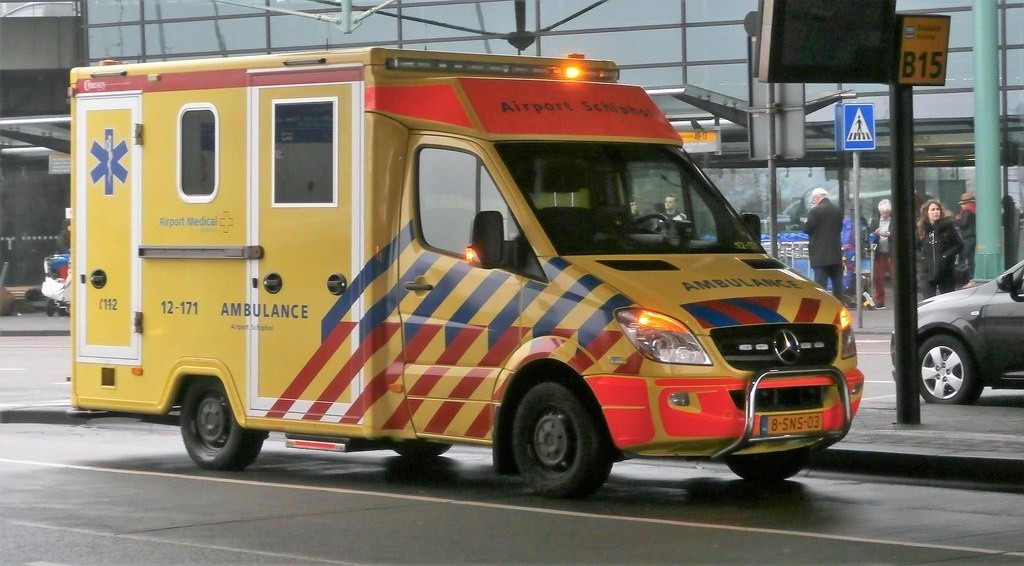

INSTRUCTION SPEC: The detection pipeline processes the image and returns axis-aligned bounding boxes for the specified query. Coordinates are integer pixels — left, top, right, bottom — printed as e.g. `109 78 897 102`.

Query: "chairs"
424 207 588 255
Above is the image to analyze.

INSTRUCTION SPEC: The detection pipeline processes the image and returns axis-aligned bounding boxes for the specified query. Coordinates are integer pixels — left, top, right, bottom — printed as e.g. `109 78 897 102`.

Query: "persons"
949 193 976 282
918 199 965 300
868 199 896 310
659 195 687 222
841 204 869 295
803 188 844 302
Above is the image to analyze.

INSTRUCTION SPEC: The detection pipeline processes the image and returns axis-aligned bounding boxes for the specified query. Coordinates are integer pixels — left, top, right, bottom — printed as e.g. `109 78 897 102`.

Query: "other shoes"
869 304 885 310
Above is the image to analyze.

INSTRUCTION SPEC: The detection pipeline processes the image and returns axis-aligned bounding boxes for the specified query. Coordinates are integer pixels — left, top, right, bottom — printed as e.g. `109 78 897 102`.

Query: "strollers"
44 254 70 317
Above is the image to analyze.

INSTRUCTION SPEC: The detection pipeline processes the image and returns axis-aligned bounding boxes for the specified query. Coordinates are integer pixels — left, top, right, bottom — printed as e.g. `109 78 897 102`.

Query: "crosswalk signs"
842 103 876 150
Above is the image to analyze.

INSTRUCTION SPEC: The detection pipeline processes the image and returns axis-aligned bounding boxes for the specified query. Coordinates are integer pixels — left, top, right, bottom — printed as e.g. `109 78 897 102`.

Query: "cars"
895 261 1024 404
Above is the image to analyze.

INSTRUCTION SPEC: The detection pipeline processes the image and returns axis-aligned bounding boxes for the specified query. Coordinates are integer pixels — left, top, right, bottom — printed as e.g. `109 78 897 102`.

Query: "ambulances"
68 47 864 499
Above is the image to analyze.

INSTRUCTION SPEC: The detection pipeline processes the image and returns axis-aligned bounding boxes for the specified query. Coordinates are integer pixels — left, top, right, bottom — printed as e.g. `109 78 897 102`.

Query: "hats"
957 193 975 205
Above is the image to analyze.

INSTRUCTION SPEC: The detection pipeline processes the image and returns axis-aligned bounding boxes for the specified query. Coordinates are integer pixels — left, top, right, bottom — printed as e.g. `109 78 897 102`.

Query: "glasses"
810 199 815 204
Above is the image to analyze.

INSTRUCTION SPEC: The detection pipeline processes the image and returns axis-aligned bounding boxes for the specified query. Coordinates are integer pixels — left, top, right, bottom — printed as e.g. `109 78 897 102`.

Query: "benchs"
3 285 45 313
